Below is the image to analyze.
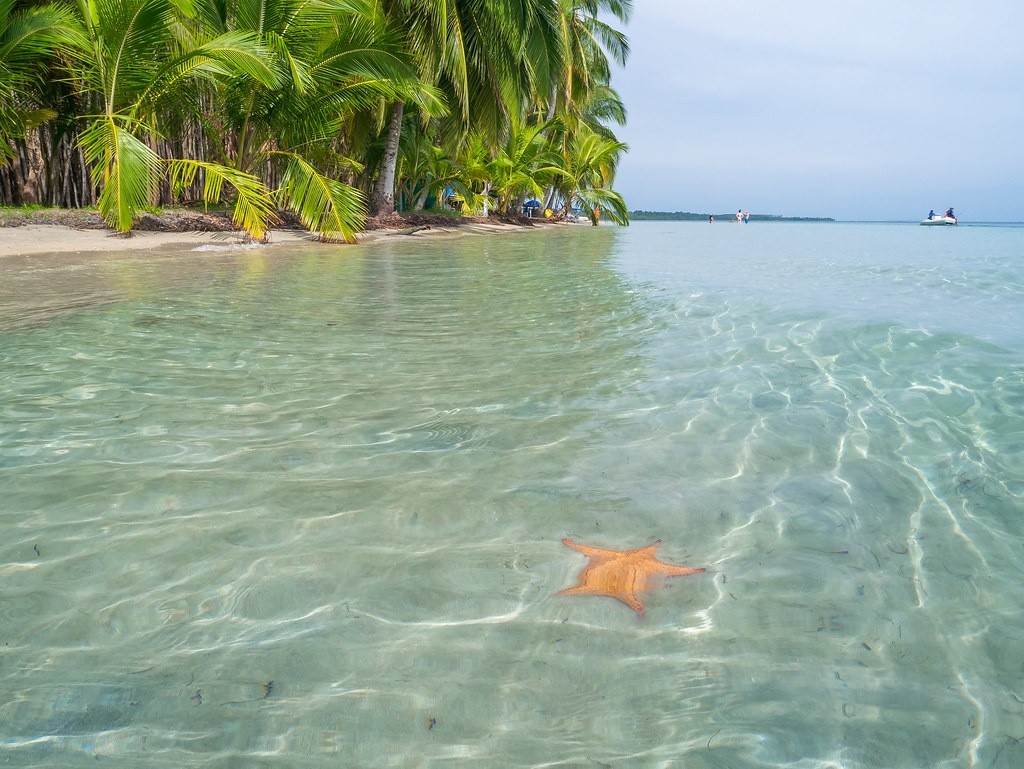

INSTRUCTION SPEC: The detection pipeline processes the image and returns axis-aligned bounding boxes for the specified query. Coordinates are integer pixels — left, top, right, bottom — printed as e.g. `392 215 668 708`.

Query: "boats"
920 213 956 226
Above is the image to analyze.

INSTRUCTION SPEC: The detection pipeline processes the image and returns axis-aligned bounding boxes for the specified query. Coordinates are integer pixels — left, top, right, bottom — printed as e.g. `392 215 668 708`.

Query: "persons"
946 208 957 227
524 207 529 217
929 210 935 220
736 209 749 224
709 214 714 223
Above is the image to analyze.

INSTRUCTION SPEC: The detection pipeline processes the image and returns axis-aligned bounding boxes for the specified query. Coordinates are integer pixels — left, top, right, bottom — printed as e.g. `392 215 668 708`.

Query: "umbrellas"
524 200 541 207
